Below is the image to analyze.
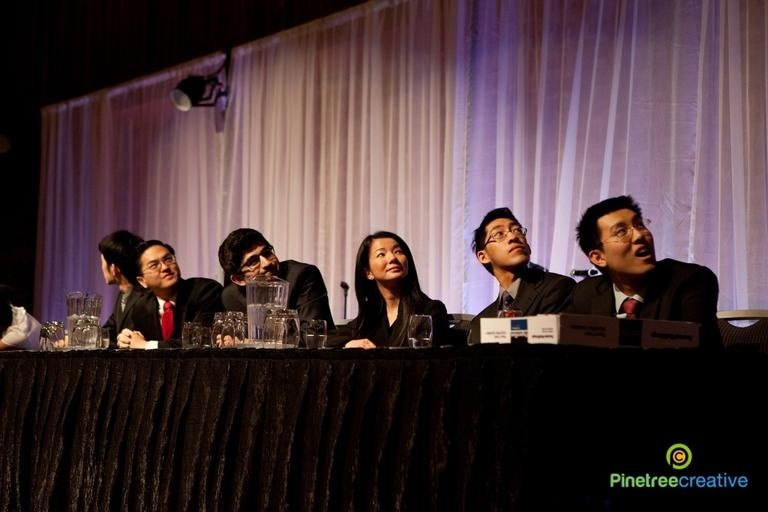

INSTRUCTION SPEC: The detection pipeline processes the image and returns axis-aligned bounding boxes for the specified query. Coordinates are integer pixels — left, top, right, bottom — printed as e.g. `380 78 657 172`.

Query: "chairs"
714 309 768 349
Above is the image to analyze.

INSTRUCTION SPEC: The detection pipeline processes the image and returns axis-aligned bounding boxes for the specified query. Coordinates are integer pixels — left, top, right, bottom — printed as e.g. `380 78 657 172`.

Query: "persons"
217 226 336 347
115 239 222 348
315 231 452 349
44 227 148 350
565 195 722 347
460 207 577 345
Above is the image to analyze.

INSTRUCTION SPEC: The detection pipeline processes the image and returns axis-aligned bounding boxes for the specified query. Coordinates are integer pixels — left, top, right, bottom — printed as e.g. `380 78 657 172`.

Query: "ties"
119 294 127 315
621 299 638 320
500 292 514 317
161 301 174 341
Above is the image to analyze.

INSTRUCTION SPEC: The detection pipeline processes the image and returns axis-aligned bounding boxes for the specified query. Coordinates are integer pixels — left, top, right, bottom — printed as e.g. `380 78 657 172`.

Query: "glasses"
478 227 527 249
597 219 652 243
138 254 176 272
237 246 275 272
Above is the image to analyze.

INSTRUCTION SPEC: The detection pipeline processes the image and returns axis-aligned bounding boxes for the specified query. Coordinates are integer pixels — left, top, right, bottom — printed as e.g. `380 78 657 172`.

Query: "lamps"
168 47 232 113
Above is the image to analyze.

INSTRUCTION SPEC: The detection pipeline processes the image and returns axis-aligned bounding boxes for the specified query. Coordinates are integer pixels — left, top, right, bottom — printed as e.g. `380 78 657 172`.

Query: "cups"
38 320 110 352
443 319 472 347
180 310 328 350
406 314 433 349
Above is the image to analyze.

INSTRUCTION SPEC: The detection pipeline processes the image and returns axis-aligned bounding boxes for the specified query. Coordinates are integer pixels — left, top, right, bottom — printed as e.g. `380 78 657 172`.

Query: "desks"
0 343 768 512
